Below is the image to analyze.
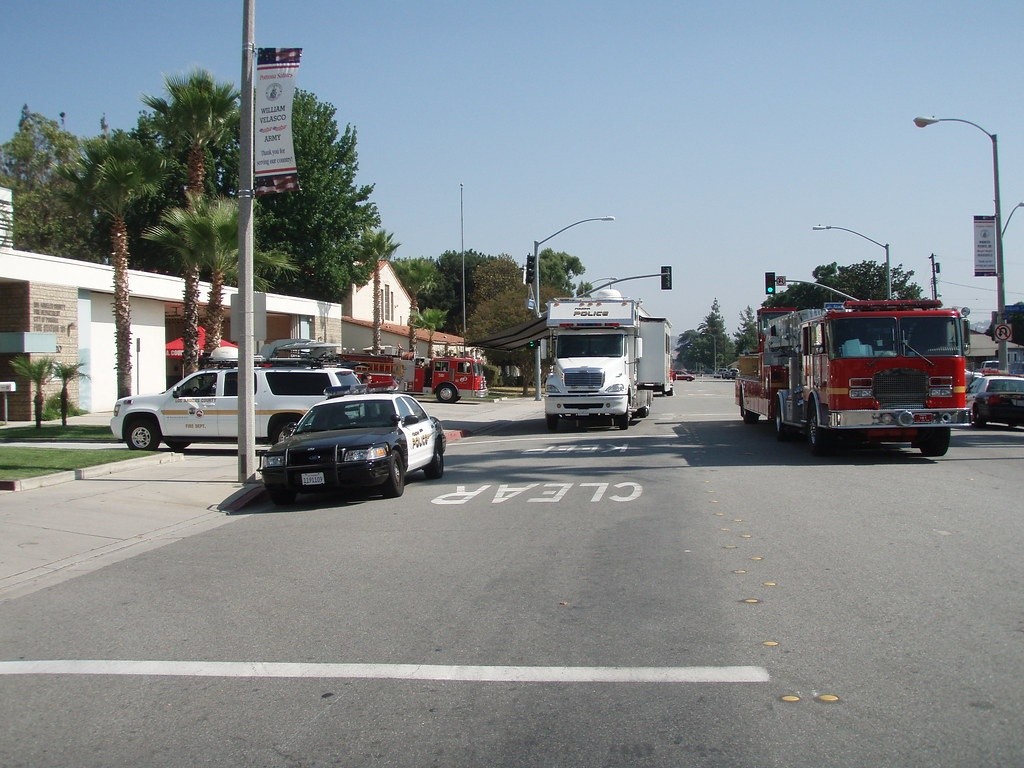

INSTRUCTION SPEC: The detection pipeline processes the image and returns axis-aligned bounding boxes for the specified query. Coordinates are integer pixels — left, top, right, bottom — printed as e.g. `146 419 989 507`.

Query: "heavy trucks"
541 288 675 429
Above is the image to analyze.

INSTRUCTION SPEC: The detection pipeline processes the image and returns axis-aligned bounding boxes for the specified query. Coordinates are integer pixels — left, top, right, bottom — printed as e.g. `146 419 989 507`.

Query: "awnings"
467 314 551 353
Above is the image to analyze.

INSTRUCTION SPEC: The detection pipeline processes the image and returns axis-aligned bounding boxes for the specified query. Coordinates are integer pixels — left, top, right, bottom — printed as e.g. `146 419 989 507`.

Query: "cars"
962 359 1024 431
672 370 695 382
259 379 447 507
686 368 740 380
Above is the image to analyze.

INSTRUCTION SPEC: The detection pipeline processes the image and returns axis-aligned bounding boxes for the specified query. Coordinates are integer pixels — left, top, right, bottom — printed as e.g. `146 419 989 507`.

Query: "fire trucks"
733 299 971 466
318 343 490 403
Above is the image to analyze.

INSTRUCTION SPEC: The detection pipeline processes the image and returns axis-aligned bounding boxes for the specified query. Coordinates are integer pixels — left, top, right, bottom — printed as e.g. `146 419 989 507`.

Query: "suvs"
110 346 380 457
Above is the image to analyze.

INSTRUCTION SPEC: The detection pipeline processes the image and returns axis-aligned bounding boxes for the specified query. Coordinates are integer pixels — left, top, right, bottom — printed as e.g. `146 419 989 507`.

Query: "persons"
354 372 372 384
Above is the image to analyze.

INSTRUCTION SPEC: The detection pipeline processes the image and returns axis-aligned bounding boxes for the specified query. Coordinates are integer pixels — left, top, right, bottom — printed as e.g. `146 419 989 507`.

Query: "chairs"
325 407 350 428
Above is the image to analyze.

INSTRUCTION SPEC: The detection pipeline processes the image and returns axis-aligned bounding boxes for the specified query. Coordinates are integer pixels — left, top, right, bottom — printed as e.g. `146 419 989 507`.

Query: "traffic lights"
524 254 537 285
528 340 534 350
764 272 776 294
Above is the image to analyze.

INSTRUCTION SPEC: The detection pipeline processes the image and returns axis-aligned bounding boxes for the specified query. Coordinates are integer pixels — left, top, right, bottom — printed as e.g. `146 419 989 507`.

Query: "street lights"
812 224 892 300
702 333 716 373
534 215 616 401
912 116 1008 373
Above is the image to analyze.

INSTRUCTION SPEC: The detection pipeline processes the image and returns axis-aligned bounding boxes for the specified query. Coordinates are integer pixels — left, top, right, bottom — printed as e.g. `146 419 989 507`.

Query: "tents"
166 326 238 379
